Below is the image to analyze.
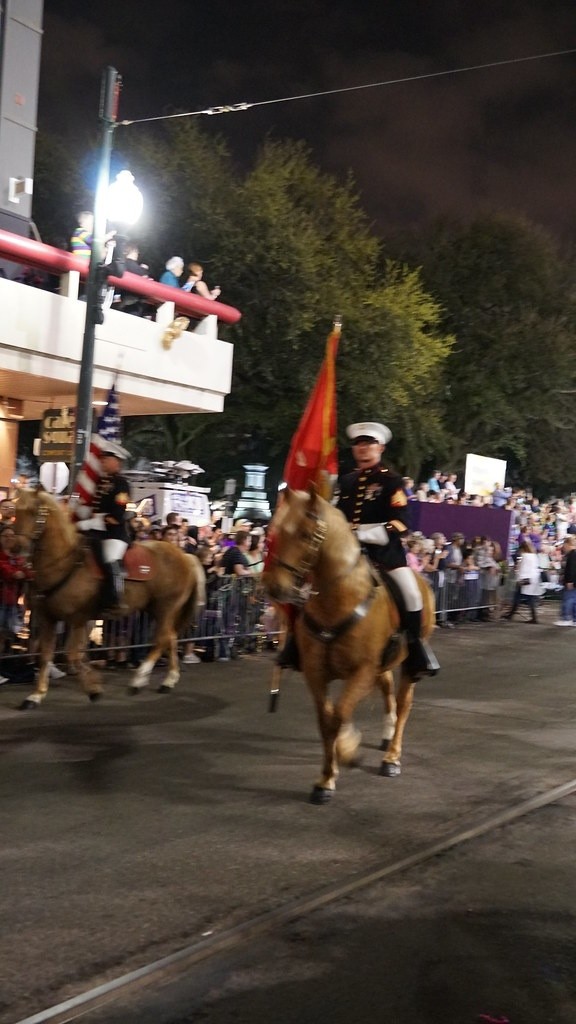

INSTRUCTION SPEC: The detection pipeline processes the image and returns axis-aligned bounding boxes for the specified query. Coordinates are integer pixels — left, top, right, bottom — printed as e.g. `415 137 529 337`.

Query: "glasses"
0 534 15 538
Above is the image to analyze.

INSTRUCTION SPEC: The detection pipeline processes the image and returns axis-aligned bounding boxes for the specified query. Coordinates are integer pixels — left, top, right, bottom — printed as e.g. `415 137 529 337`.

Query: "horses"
7 479 206 710
261 480 435 806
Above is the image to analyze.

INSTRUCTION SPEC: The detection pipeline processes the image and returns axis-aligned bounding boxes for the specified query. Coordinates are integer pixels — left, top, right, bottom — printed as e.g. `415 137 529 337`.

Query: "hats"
411 531 425 540
98 440 132 462
249 527 265 535
150 515 161 523
235 519 253 528
346 422 392 446
149 525 161 534
451 533 466 540
227 526 237 534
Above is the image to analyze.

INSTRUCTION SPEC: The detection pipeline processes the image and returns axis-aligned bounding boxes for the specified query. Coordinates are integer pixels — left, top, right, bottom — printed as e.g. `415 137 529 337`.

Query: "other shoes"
48 661 66 679
553 620 576 626
0 676 8 684
183 653 202 664
523 619 537 623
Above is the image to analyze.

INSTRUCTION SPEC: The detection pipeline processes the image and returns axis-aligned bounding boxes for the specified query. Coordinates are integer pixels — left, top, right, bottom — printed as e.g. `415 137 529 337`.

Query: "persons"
75 451 131 610
0 497 109 683
401 470 576 626
70 211 220 332
336 422 427 682
115 513 279 670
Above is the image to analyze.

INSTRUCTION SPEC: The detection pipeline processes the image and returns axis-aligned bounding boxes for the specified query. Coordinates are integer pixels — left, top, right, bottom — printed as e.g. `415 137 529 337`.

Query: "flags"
264 335 336 618
75 385 121 502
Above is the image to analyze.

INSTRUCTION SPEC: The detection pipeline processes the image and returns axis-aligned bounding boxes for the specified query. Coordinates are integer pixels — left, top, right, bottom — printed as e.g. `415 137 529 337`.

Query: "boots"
102 560 130 618
400 608 428 681
